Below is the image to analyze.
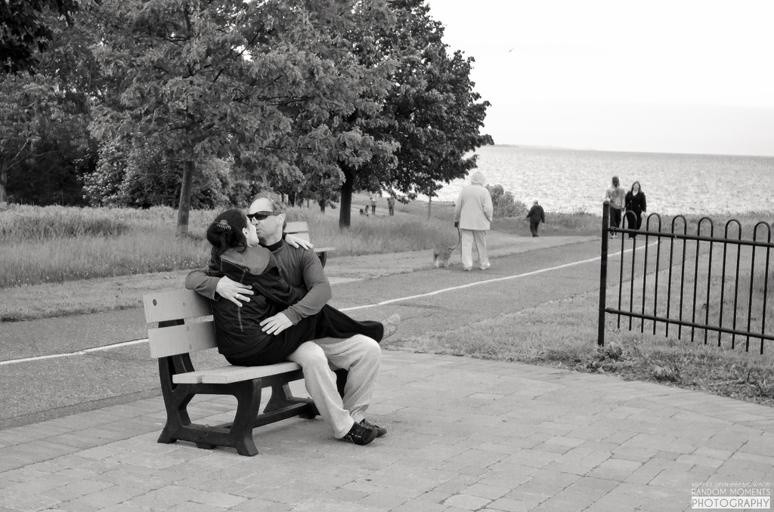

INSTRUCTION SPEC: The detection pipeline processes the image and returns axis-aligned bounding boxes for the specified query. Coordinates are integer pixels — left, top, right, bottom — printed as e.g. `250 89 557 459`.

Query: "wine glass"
235 293 239 299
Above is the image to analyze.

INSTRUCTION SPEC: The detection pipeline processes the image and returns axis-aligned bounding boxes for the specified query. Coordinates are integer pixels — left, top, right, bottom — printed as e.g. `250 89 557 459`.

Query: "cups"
247 211 277 222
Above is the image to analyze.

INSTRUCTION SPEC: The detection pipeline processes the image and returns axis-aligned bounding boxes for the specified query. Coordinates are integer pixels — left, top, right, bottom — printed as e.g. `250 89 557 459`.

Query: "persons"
603 175 625 239
355 194 396 217
624 181 646 240
451 170 493 272
204 208 402 366
183 190 388 446
526 198 545 237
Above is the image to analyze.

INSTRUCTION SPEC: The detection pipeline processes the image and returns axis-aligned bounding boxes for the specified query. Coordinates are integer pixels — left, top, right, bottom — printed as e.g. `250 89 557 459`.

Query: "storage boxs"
284 221 336 268
143 289 348 457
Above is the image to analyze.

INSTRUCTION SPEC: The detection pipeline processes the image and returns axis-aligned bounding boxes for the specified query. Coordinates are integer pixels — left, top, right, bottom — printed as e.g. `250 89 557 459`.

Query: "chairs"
342 423 377 445
379 314 400 339
360 421 387 437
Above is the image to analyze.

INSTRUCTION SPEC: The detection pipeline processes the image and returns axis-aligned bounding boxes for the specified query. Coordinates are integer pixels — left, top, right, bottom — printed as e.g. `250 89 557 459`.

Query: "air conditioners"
433 248 453 269
